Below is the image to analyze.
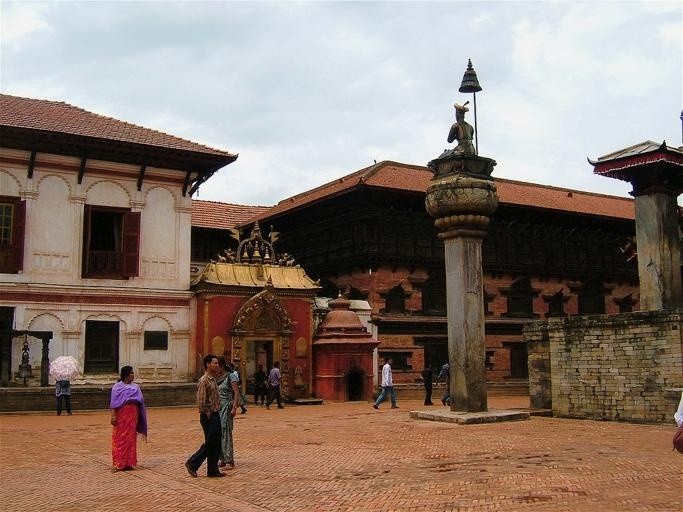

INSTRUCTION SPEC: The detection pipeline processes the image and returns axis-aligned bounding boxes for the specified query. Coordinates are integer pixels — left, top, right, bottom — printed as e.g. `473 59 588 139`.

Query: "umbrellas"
48 355 80 382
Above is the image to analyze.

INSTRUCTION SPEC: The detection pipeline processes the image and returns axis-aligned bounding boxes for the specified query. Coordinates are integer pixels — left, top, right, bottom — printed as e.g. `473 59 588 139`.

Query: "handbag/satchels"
230 397 244 409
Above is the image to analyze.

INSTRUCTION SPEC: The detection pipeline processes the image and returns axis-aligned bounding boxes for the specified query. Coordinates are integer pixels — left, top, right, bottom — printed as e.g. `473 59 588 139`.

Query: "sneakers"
186 462 197 477
207 462 234 477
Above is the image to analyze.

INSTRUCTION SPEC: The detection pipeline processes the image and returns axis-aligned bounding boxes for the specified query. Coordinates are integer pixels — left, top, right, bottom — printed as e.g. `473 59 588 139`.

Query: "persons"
184 355 227 479
419 361 434 405
54 378 73 416
438 104 476 157
108 366 148 473
264 361 285 410
674 391 683 427
228 363 248 414
210 355 239 471
435 358 450 406
373 356 400 409
251 364 266 404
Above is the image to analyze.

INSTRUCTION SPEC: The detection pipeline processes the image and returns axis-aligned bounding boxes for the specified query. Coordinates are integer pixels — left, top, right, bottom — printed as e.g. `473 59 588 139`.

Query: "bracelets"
233 406 237 409
